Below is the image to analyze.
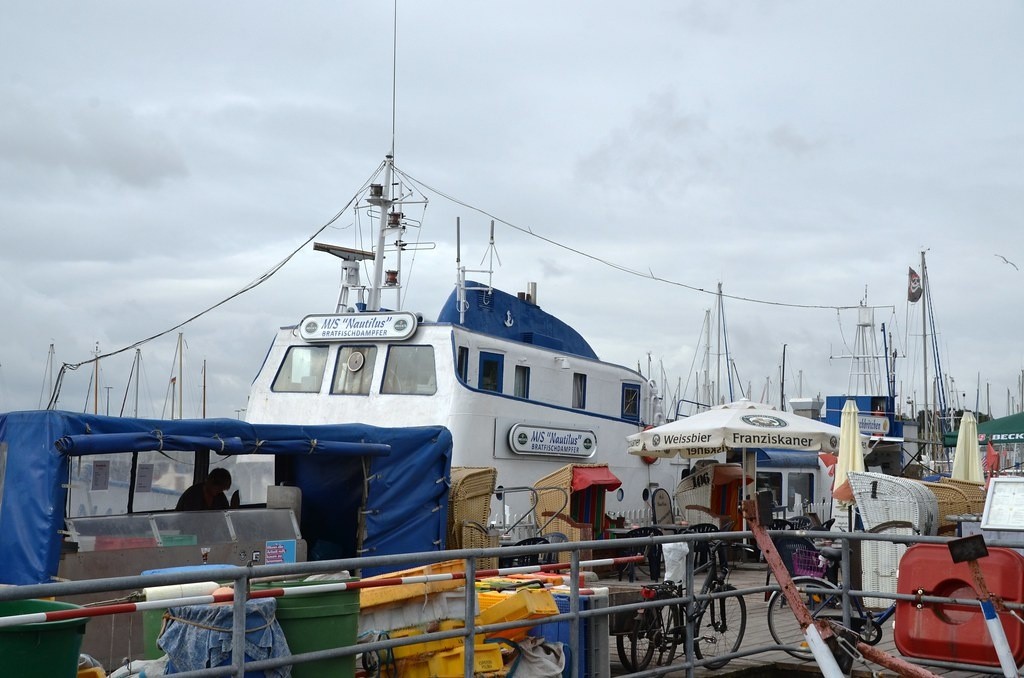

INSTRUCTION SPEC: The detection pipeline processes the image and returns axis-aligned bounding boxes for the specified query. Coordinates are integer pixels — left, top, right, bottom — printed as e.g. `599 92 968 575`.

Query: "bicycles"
765 527 923 662
630 540 747 678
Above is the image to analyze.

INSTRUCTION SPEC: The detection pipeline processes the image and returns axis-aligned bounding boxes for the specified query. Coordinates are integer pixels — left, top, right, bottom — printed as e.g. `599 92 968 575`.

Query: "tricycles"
586 582 660 671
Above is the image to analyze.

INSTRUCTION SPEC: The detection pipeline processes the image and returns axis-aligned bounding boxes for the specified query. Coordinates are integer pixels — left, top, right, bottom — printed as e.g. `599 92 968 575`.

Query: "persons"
174 468 232 510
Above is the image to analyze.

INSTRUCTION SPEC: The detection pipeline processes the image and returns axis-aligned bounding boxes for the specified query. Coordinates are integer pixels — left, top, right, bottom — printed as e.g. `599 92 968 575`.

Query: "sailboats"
638 248 1024 475
34 330 208 496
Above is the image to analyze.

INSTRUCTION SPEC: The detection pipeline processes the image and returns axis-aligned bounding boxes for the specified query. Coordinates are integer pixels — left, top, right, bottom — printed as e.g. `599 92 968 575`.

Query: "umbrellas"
942 412 1024 446
951 410 985 484
625 397 872 545
833 397 865 534
